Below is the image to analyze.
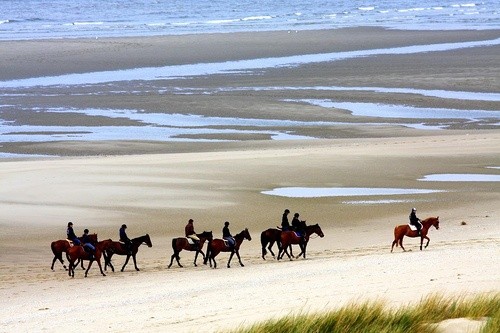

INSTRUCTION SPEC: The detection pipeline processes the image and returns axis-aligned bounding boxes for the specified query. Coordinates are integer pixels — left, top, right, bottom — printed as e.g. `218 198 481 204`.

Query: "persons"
292 213 307 243
409 207 422 235
83 228 97 259
282 209 291 231
68 221 82 245
186 219 201 251
120 224 134 255
223 221 238 253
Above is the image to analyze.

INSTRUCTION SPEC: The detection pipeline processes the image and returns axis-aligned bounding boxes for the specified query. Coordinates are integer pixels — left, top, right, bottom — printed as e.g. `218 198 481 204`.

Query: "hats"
412 208 416 210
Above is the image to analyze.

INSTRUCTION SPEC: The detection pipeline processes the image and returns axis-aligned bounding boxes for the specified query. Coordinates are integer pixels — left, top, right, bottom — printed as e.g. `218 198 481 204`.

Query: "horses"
391 215 439 251
277 222 324 260
51 232 98 271
168 230 214 268
102 233 152 271
202 227 251 267
66 238 116 277
260 219 307 260
